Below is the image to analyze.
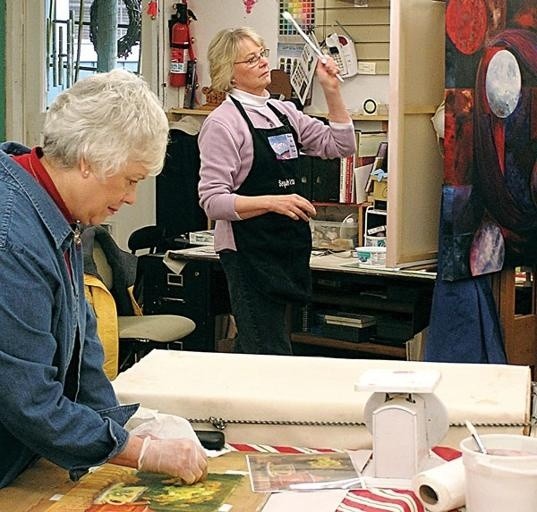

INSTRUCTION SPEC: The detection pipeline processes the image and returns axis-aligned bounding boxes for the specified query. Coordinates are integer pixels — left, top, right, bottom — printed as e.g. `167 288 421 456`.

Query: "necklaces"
28 157 81 246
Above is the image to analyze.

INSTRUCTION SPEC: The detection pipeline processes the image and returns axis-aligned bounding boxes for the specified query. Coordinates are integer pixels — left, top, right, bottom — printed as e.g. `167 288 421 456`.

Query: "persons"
196 25 357 355
1 67 207 496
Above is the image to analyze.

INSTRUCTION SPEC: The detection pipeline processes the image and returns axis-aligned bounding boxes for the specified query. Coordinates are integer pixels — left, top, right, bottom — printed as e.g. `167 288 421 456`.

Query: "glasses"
232 49 269 69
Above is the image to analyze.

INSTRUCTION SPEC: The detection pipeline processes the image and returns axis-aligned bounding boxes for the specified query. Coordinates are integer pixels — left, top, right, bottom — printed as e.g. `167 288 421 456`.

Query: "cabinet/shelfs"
137 255 230 362
167 108 389 246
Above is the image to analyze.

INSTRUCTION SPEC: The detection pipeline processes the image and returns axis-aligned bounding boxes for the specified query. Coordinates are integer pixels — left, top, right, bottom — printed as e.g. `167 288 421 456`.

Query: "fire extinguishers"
168 4 197 87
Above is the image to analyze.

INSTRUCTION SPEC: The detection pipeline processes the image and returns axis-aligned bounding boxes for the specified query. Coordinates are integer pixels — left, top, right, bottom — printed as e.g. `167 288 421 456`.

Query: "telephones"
326 34 358 80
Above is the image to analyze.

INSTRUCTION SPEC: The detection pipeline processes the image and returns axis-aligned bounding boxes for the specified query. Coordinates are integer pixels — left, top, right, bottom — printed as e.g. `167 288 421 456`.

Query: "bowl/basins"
355 247 387 265
367 236 387 247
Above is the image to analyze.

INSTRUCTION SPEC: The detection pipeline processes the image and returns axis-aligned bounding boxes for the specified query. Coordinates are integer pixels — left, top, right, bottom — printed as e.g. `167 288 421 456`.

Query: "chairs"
79 225 196 372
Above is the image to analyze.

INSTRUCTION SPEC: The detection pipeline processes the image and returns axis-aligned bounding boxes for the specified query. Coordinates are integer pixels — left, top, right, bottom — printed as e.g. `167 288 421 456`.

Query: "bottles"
345 218 358 246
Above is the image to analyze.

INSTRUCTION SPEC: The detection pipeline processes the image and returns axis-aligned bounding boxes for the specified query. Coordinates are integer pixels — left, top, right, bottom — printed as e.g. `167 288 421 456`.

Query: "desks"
170 241 532 356
1 346 537 512
